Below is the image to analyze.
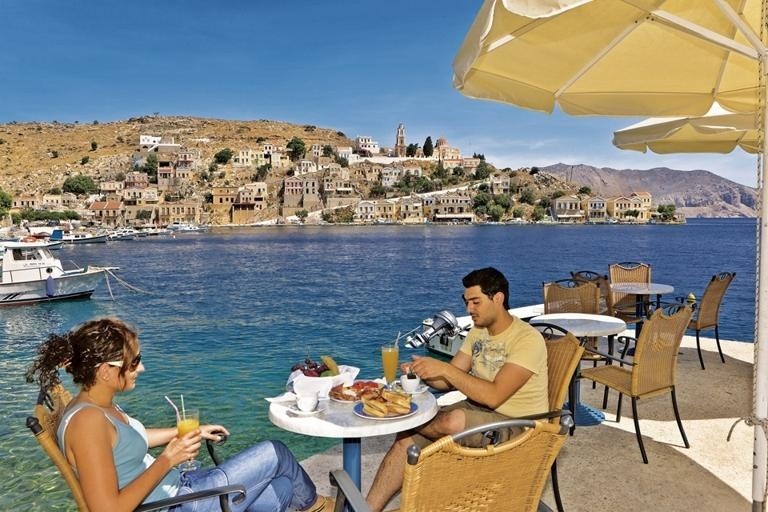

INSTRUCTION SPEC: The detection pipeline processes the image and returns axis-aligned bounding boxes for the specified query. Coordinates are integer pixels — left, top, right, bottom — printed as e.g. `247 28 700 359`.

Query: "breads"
363 390 414 417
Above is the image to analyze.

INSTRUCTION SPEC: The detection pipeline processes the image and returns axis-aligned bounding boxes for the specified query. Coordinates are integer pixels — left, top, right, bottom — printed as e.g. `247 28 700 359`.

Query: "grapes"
292 356 327 376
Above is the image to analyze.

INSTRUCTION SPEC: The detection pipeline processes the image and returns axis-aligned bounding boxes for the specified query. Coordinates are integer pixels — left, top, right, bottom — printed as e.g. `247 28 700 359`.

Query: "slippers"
303 495 337 512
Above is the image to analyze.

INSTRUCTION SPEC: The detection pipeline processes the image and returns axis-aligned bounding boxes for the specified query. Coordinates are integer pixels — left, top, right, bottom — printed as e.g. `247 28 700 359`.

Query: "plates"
392 384 429 396
285 401 329 416
354 402 419 420
329 382 388 403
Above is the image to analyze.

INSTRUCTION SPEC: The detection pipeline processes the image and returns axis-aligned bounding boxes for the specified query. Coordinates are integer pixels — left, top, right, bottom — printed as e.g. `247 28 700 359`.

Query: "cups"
400 374 420 392
296 389 320 411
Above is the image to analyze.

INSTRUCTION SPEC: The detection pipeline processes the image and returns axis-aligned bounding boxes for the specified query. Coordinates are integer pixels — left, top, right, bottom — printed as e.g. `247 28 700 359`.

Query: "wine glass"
178 409 200 471
380 345 399 393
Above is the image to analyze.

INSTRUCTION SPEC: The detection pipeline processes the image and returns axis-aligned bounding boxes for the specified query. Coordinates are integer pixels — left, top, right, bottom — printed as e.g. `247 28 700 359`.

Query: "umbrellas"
612 101 768 155
451 0 768 511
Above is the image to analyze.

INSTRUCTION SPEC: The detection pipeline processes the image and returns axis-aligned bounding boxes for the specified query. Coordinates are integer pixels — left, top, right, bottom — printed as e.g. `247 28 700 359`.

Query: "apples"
306 371 319 377
320 371 335 377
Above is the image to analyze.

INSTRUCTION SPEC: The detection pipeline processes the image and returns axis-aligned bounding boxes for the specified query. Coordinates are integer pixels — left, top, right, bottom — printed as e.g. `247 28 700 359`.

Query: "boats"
0 221 212 250
0 241 119 306
404 294 608 357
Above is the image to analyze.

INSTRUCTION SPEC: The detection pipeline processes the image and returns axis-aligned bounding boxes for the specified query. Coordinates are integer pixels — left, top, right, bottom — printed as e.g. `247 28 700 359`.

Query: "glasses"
94 353 143 373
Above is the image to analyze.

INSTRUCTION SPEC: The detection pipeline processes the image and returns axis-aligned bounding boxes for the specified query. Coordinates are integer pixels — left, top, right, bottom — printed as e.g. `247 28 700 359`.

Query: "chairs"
25 374 246 512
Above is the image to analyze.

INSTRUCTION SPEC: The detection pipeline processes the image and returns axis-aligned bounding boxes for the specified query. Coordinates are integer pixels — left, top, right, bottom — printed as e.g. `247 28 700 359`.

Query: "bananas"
320 355 339 373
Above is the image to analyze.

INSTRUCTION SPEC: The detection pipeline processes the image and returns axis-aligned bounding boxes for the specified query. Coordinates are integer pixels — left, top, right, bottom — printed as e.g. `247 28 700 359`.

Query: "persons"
365 267 549 512
23 314 337 512
